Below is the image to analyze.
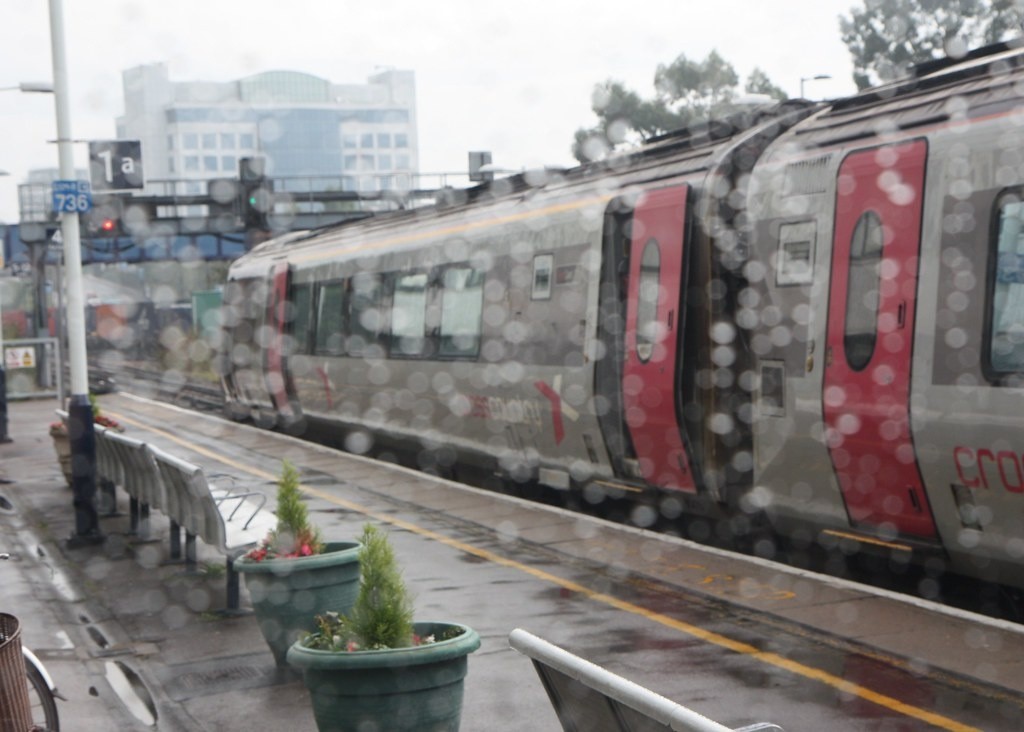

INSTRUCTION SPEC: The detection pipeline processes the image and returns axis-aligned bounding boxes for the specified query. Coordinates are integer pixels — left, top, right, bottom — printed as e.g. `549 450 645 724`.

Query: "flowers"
300 520 465 653
241 456 323 562
51 412 122 432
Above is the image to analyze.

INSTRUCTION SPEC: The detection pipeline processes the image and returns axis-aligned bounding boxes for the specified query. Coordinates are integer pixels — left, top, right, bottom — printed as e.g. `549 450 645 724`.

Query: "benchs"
508 628 785 731
93 423 279 616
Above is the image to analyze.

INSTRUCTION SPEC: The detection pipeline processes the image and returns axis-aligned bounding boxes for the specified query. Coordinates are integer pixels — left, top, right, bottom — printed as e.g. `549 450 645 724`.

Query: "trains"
224 32 1024 615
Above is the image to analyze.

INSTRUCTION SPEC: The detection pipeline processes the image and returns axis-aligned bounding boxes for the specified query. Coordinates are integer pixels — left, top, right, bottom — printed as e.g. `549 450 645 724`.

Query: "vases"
232 538 366 667
285 620 482 732
48 432 74 489
